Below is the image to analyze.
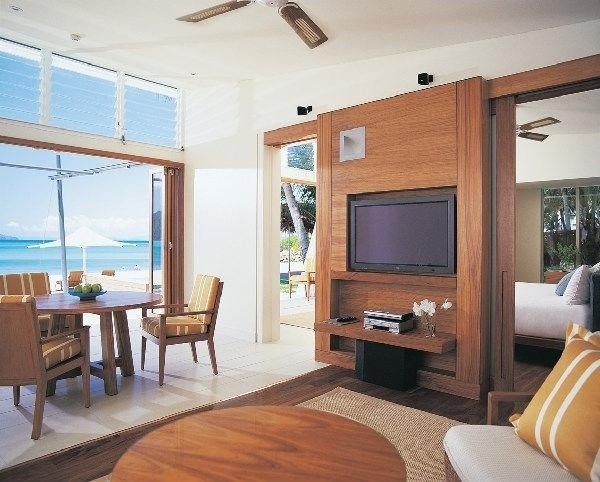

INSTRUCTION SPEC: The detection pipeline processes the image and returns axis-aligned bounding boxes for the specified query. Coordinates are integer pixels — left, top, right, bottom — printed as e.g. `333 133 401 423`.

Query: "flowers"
412 297 454 321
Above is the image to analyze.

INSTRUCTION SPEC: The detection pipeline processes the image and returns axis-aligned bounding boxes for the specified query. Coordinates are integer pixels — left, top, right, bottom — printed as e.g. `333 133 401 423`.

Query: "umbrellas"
26 229 137 281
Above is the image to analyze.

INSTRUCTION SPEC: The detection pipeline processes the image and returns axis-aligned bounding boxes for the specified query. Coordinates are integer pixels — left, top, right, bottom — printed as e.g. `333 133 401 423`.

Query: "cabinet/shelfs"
314 270 457 383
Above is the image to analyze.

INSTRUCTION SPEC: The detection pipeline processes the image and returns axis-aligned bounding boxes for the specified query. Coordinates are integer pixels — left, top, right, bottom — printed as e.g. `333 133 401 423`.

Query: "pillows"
508 322 600 482
556 268 576 297
563 262 599 305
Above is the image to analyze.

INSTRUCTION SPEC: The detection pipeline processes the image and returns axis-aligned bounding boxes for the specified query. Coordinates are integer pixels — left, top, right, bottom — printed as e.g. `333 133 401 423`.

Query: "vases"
423 321 436 338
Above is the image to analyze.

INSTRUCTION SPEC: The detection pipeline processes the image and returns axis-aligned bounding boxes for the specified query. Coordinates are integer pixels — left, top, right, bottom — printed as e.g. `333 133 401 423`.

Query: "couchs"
442 322 600 482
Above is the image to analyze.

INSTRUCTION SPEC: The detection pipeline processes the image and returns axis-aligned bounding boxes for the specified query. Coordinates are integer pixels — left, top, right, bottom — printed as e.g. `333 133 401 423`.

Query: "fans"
175 0 330 51
515 114 561 143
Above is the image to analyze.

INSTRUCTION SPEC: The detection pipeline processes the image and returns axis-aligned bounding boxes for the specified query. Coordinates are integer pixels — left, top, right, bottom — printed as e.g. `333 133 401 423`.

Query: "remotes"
337 316 356 322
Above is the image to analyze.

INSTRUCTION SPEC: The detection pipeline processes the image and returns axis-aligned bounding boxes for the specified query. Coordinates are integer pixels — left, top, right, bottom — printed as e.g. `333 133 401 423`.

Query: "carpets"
1 364 526 481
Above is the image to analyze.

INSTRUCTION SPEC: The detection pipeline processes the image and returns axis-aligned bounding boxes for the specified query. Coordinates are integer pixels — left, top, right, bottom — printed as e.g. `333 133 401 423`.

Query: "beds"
515 261 600 351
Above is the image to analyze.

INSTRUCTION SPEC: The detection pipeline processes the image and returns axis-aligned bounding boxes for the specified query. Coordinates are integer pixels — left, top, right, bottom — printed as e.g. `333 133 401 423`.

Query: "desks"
31 291 162 396
112 404 408 481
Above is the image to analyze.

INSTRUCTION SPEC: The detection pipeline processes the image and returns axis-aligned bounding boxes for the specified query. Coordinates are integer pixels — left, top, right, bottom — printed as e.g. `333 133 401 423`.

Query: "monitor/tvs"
350 195 456 275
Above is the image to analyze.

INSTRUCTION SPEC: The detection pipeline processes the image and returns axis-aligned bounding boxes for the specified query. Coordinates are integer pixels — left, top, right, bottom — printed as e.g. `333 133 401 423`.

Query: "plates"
66 289 106 301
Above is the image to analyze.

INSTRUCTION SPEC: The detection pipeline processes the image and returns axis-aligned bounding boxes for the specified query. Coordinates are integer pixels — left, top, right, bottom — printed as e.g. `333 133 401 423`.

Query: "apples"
74 283 102 293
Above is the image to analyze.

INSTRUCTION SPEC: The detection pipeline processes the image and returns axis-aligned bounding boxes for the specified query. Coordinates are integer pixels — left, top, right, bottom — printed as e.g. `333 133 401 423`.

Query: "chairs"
140 274 224 385
0 270 74 338
0 294 91 439
288 256 315 301
55 270 87 290
